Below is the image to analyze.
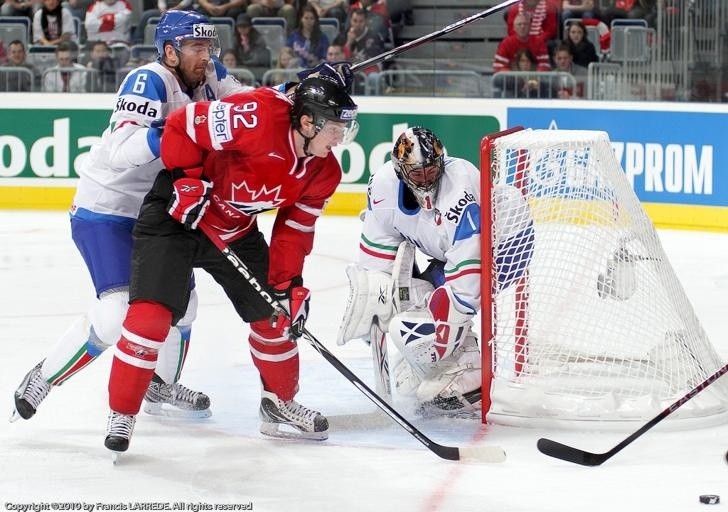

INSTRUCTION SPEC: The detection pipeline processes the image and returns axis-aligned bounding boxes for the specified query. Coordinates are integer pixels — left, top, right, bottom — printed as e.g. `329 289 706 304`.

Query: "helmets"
390 125 446 210
154 7 221 59
293 77 360 146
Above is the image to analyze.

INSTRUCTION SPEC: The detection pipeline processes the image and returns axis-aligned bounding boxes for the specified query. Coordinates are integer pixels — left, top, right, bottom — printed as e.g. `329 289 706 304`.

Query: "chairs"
562 19 602 63
316 17 339 46
132 45 159 62
607 20 649 63
144 18 163 47
210 17 236 57
0 16 30 54
251 18 286 69
128 0 144 27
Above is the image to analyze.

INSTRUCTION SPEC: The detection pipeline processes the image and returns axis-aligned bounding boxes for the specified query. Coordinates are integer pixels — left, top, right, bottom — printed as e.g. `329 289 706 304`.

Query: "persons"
512 48 557 98
333 9 384 79
550 46 588 99
507 0 556 39
88 40 109 70
560 22 599 69
230 12 270 68
273 47 307 86
220 48 261 89
493 14 552 72
43 41 87 92
322 45 343 63
0 1 31 18
0 39 42 92
31 0 78 53
137 0 389 27
358 127 535 417
287 7 330 69
104 77 358 452
12 10 243 421
85 0 133 43
562 0 594 20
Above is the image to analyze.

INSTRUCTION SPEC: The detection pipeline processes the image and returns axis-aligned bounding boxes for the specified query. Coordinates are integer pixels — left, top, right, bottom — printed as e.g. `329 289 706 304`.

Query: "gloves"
165 175 214 230
268 276 315 345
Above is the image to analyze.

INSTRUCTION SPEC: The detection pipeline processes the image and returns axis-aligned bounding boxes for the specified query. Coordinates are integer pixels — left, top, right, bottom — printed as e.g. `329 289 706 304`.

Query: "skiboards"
535 363 728 466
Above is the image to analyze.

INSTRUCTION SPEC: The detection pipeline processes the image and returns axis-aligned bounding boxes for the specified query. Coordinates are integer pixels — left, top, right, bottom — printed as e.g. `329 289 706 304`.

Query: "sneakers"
144 380 212 411
258 389 330 433
102 409 136 452
16 366 54 420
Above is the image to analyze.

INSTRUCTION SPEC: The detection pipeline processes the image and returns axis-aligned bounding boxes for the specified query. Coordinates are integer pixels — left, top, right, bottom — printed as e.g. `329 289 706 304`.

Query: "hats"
234 14 253 27
